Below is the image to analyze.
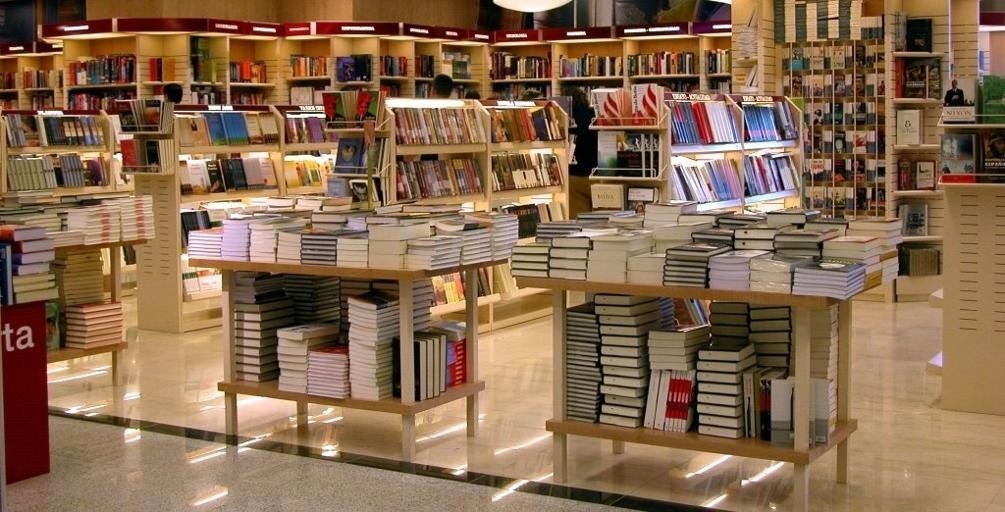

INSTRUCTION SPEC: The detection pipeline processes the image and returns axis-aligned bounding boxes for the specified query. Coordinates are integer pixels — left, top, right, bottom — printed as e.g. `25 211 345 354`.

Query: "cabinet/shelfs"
586 89 807 216
495 36 732 180
380 96 568 336
120 104 339 337
749 3 946 311
288 33 488 101
2 52 66 113
177 260 505 461
56 35 282 112
512 274 858 511
1 107 141 299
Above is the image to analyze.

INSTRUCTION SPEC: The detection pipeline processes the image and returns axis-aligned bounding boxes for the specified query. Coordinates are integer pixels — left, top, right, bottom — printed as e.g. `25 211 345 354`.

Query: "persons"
944 79 965 106
987 135 1004 160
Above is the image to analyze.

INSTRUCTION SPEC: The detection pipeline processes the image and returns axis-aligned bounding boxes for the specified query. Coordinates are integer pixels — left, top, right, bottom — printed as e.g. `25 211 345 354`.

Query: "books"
66 301 123 349
979 73 1005 124
939 133 978 184
897 16 944 275
0 38 905 312
230 270 468 405
564 295 837 458
942 74 978 123
983 132 1005 183
